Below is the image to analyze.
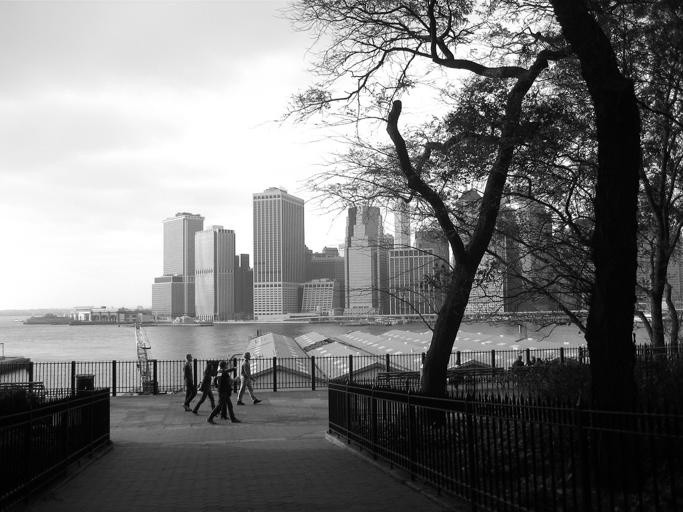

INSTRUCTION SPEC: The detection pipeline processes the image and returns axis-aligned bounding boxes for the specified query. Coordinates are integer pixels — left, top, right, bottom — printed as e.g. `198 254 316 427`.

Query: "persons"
182 352 262 425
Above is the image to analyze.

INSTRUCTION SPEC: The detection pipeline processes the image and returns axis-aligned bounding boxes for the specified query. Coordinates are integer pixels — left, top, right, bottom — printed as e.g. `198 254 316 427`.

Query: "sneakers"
254 399 261 404
182 405 240 423
237 401 244 405
